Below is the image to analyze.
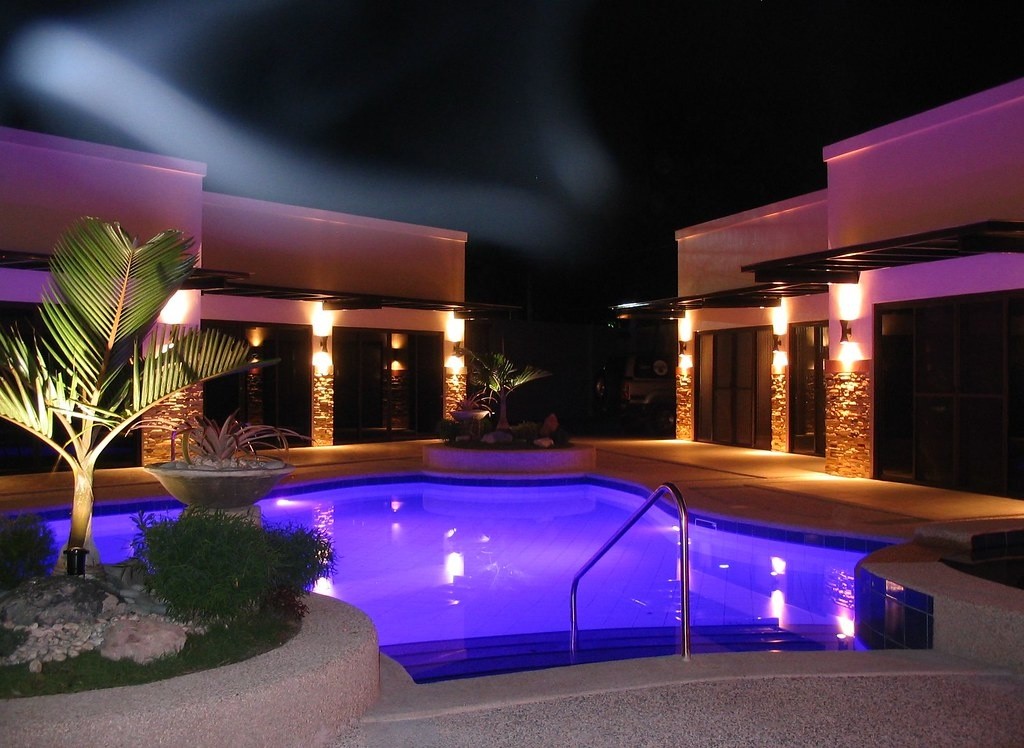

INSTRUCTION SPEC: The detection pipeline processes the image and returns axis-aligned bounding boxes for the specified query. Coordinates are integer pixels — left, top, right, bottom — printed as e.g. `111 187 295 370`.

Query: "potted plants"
123 411 319 530
441 384 498 425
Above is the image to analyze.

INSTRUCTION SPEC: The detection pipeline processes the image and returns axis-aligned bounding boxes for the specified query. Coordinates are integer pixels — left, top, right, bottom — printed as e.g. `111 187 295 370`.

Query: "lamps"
677 340 688 357
453 340 462 359
838 318 853 345
321 335 331 355
63 546 91 577
772 333 783 354
